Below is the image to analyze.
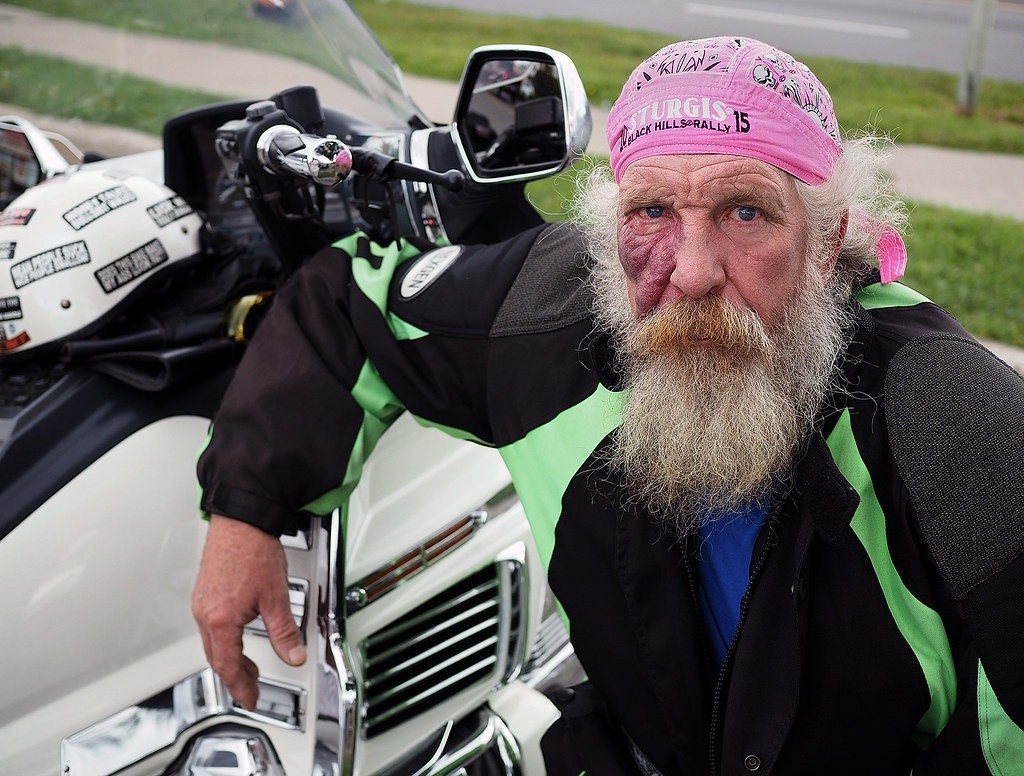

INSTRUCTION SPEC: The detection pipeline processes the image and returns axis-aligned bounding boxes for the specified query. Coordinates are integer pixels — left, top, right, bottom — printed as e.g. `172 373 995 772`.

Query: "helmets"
0 172 202 353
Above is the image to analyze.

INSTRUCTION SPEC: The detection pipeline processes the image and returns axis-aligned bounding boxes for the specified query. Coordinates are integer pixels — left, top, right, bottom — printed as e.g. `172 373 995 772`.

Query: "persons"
191 36 1024 776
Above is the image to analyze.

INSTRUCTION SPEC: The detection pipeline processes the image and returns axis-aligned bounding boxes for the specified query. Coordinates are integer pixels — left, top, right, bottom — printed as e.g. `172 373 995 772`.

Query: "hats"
605 37 843 186
